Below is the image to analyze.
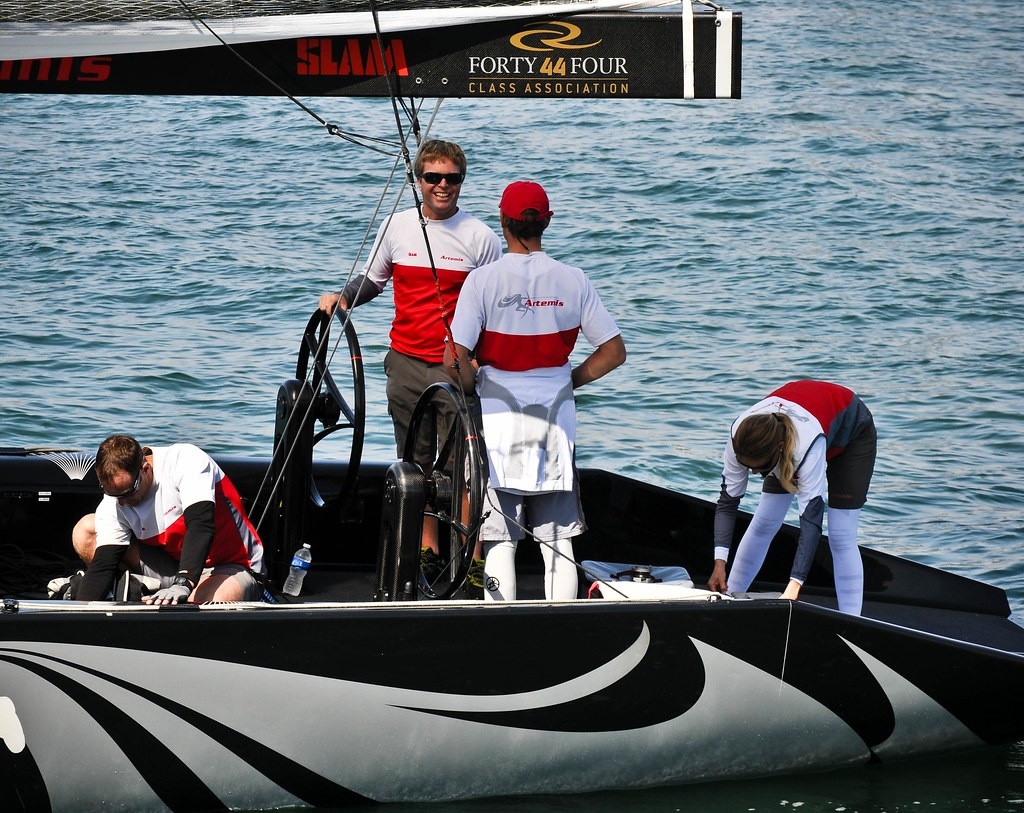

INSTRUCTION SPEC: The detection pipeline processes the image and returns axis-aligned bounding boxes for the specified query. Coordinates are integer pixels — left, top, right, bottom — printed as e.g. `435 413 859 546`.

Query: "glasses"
101 468 142 499
418 172 464 185
735 448 777 470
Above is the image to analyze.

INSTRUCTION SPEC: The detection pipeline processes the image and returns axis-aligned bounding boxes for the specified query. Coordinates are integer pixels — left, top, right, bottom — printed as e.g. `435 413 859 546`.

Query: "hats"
498 180 554 221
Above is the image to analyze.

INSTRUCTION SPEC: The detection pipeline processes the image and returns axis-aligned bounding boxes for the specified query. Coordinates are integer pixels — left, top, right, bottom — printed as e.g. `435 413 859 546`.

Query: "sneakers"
418 546 447 584
459 558 485 600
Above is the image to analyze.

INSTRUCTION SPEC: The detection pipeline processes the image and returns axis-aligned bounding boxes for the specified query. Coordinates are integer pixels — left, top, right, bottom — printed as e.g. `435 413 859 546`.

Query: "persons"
319 139 502 601
72 435 264 605
444 181 626 600
707 379 878 618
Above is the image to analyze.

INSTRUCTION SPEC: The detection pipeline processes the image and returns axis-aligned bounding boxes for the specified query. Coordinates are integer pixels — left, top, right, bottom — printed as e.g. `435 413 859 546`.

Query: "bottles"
283 543 312 597
